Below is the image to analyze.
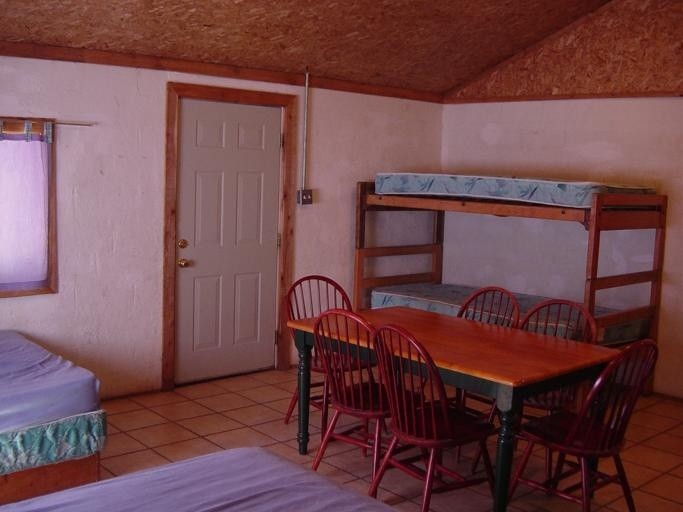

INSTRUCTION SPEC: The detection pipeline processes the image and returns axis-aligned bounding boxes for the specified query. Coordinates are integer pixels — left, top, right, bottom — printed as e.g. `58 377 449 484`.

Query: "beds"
0 332 106 512
351 167 670 397
0 444 399 512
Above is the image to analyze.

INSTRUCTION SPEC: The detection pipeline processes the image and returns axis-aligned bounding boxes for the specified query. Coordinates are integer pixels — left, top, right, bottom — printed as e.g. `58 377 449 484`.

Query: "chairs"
283 273 658 512
